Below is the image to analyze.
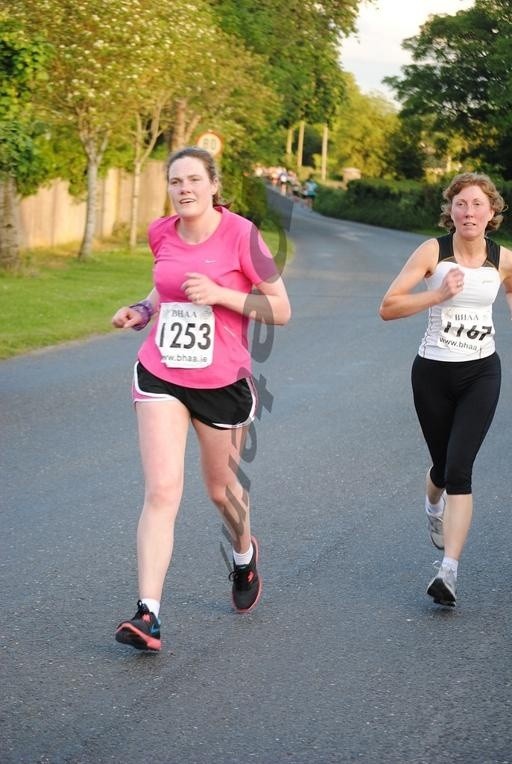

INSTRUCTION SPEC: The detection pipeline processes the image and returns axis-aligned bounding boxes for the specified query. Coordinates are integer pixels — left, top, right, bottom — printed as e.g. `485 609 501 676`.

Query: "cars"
254 166 319 199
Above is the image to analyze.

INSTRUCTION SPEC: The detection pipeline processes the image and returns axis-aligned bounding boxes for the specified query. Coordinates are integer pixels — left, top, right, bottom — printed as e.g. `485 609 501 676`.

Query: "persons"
111 144 294 656
249 161 320 212
378 169 511 607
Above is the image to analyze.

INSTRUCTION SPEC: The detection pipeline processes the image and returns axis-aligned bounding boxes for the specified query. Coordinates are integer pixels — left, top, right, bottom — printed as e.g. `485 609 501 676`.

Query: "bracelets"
127 299 155 331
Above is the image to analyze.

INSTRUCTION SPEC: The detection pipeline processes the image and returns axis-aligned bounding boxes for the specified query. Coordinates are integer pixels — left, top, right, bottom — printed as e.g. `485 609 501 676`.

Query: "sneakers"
116 606 162 652
228 540 262 612
426 566 457 607
425 502 443 550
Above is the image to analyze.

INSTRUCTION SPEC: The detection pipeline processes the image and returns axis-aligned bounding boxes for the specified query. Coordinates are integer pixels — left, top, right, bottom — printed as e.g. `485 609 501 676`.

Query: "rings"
456 280 460 286
196 292 200 299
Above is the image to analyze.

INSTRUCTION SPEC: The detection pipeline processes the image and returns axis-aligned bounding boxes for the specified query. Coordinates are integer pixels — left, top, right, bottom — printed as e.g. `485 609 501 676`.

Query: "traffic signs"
196 129 224 158
